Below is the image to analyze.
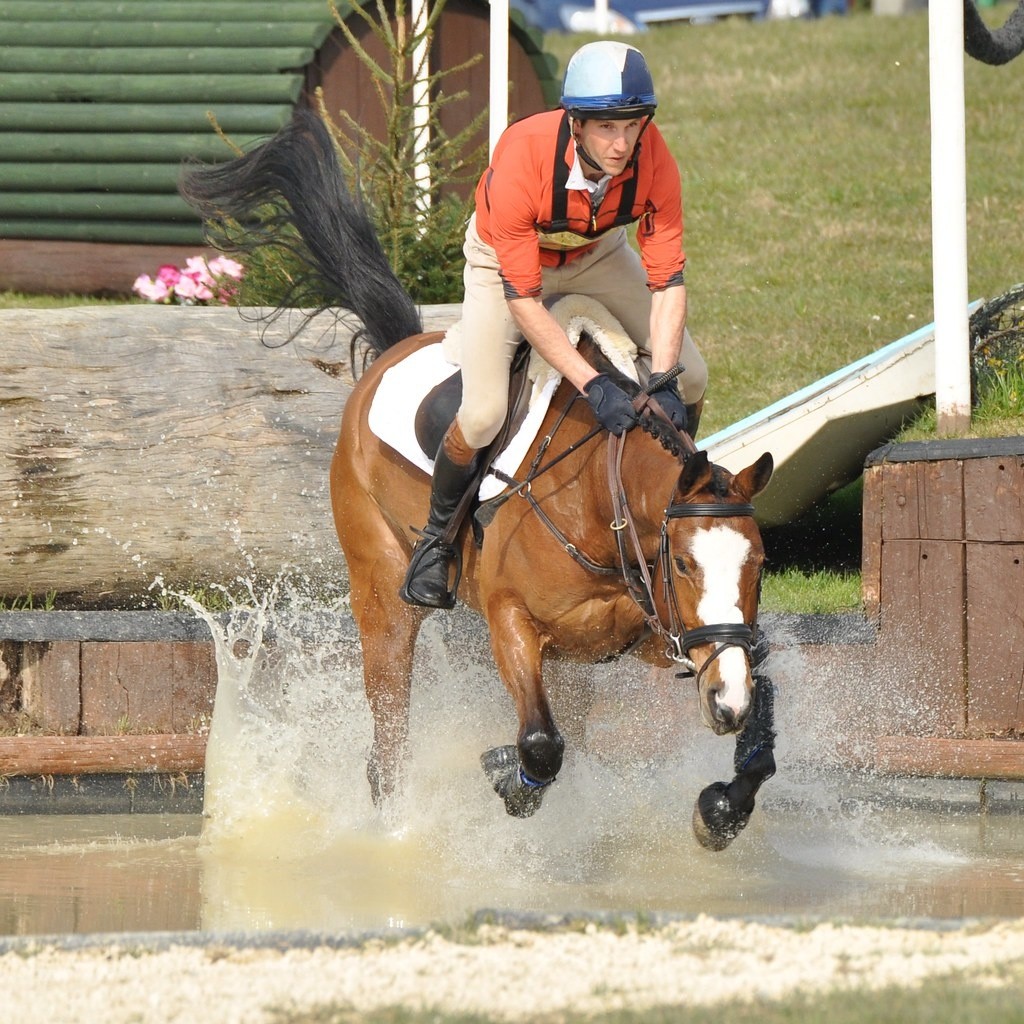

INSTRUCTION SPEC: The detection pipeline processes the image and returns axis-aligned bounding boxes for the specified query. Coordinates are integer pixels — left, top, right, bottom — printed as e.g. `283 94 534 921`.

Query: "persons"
406 41 708 606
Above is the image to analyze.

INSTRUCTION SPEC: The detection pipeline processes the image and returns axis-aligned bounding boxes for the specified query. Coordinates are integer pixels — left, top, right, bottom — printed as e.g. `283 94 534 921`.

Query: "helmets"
560 41 657 111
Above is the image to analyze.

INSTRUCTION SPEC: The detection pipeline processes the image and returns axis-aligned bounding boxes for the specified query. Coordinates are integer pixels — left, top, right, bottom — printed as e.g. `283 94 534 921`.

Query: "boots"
405 432 480 609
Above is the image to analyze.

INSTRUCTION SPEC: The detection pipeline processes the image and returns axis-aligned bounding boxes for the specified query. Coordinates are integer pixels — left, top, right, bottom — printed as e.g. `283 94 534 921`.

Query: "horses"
174 107 778 852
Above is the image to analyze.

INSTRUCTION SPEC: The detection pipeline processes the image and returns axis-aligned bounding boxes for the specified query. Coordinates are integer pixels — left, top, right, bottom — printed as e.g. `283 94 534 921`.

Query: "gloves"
576 372 640 437
640 377 687 456
